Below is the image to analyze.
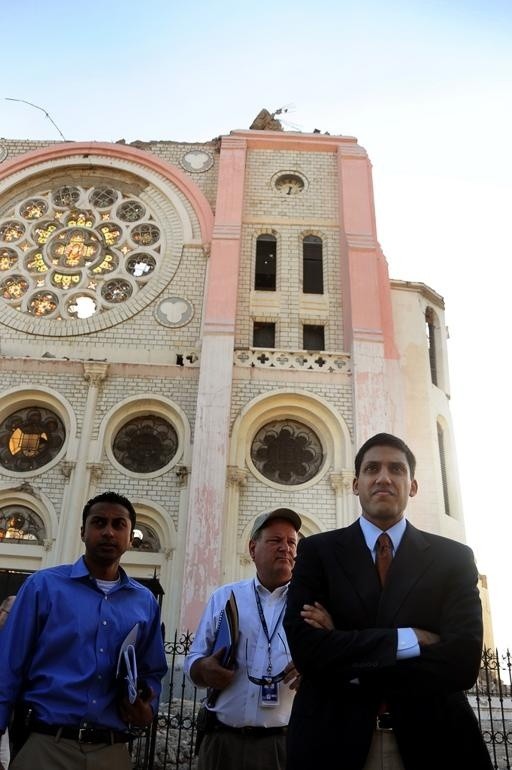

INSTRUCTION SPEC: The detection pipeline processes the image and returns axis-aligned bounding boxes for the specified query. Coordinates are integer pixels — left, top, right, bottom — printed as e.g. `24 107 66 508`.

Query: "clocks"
270 170 309 198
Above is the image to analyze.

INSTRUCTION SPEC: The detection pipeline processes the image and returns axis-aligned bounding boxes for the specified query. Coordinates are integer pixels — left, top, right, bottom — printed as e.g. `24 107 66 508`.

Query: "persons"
181 508 303 769
282 431 495 769
0 489 169 770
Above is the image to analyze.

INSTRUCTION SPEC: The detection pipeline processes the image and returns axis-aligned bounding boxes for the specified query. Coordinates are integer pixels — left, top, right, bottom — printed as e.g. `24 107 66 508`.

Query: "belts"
375 713 395 733
214 720 289 737
30 718 139 746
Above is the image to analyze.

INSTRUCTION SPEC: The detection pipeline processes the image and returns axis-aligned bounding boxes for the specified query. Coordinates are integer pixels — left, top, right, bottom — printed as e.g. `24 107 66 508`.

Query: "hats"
250 508 302 539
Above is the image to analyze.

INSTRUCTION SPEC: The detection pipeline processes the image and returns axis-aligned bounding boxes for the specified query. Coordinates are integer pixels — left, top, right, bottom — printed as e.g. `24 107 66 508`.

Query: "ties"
376 533 393 585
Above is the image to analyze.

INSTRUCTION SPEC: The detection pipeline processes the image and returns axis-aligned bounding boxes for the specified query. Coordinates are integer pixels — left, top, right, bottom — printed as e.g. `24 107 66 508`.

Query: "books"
209 608 232 693
